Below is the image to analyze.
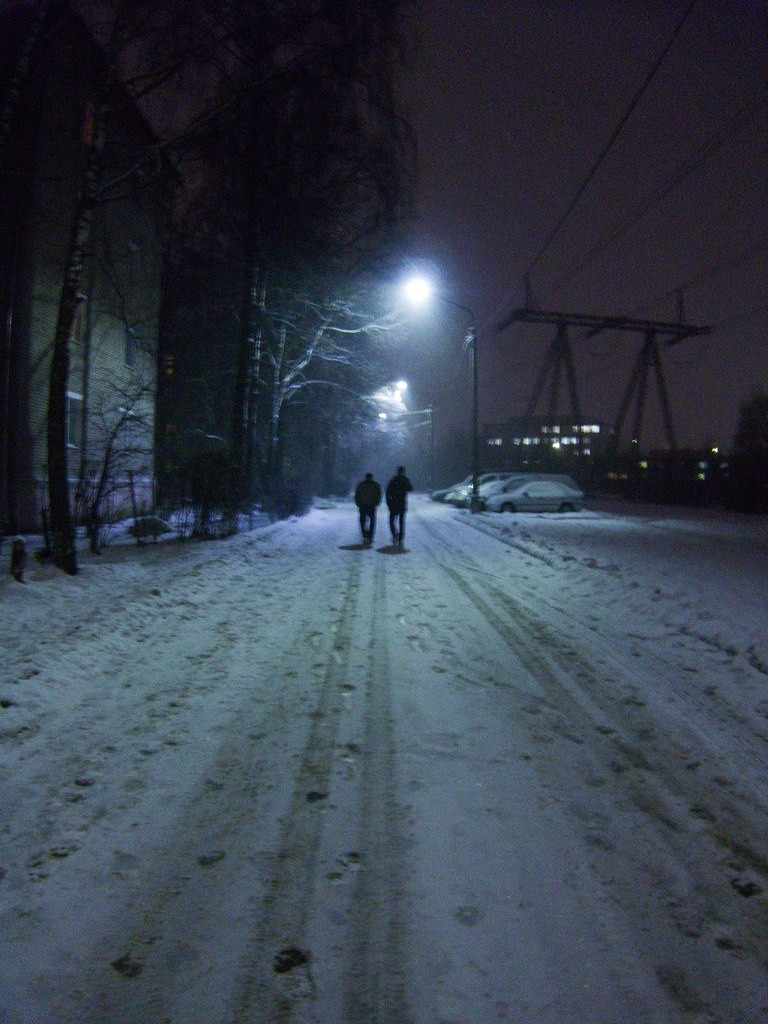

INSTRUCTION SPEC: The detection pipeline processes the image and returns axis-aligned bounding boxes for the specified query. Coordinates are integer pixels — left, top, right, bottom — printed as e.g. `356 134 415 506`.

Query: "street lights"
405 278 487 519
397 376 434 498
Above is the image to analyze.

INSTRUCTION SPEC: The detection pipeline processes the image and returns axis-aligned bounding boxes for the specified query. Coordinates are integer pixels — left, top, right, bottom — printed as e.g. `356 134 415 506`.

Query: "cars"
486 481 585 514
431 472 579 511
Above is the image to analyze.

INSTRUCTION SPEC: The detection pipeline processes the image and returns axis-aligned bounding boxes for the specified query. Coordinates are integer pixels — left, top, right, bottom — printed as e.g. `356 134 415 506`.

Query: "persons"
354 472 382 545
385 465 414 546
